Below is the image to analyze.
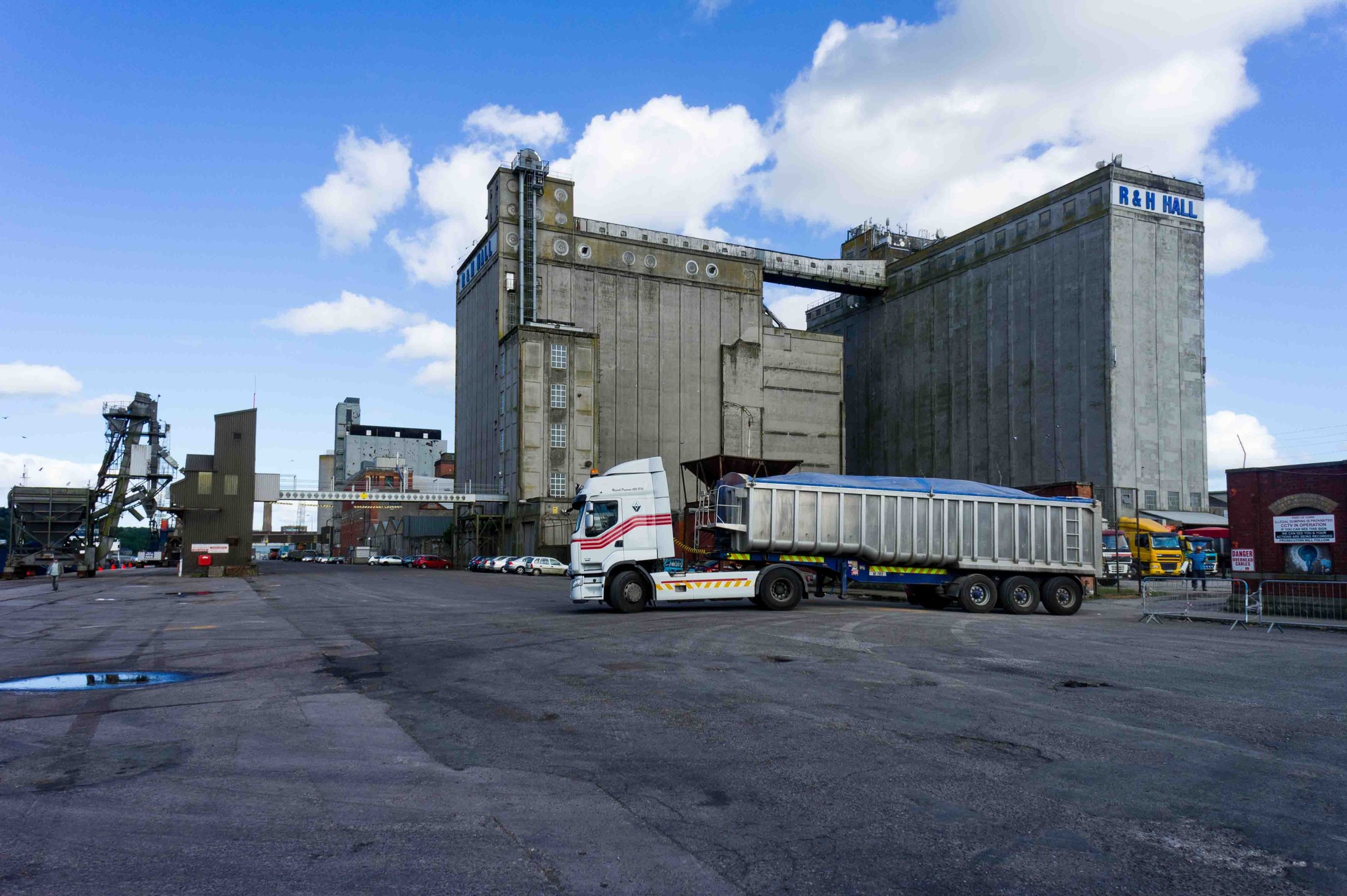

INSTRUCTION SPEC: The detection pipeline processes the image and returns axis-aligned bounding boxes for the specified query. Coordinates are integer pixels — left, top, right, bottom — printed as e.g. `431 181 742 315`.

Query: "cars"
468 556 495 571
525 556 569 578
505 556 539 575
401 555 417 568
377 555 402 566
412 555 453 570
263 557 267 560
303 556 345 564
490 556 520 573
366 555 384 565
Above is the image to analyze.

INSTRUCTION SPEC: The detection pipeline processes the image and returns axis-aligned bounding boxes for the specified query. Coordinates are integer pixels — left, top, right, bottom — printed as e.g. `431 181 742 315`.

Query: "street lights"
307 514 313 550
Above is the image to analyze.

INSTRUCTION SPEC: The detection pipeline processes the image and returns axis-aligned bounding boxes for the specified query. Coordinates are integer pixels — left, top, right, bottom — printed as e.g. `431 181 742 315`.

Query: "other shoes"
52 589 57 591
1193 588 1197 590
1203 588 1207 591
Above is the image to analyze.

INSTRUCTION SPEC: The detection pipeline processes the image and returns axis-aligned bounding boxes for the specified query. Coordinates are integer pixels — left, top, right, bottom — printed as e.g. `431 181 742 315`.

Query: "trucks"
1099 519 1132 587
268 550 288 560
1179 533 1217 577
136 549 163 568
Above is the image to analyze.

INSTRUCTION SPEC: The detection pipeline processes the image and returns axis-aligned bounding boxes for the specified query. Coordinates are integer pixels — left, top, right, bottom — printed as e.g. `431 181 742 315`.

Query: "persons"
1188 546 1208 591
47 557 64 592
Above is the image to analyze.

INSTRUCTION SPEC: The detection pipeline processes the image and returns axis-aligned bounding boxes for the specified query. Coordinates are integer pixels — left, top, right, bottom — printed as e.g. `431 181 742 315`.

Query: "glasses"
54 559 58 560
1197 549 1202 551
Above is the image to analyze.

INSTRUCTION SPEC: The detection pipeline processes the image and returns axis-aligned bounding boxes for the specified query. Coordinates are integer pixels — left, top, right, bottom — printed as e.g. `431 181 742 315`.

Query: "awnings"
1137 510 1229 527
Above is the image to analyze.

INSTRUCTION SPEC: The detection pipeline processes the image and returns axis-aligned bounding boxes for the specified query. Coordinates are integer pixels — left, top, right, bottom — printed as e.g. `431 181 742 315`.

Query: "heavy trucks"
1117 516 1183 581
562 452 1103 617
280 550 315 562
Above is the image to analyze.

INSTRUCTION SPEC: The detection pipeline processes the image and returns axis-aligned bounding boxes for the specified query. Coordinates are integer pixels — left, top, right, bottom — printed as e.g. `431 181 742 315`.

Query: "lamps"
451 234 485 269
